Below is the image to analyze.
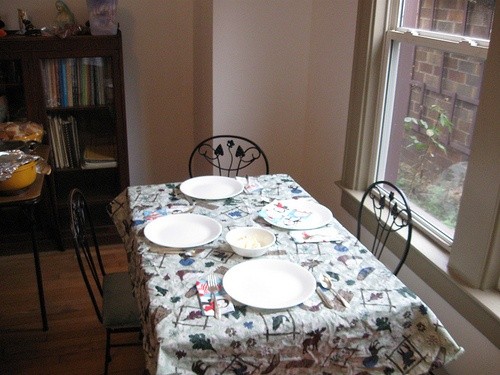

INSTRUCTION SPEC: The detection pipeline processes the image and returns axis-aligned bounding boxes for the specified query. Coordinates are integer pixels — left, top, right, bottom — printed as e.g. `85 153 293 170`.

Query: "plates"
143 213 222 249
221 257 317 310
179 176 244 201
262 200 333 231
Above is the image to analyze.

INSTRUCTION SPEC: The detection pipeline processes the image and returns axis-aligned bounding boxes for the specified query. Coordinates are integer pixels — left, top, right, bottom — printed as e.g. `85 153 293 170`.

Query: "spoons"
318 274 351 309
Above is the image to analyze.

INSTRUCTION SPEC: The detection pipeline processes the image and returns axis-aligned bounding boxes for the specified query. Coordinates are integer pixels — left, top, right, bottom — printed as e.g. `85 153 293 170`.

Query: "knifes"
149 245 215 253
184 195 193 205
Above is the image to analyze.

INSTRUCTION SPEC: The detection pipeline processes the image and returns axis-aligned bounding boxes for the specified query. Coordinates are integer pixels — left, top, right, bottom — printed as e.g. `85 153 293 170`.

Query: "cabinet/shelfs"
0 22 132 252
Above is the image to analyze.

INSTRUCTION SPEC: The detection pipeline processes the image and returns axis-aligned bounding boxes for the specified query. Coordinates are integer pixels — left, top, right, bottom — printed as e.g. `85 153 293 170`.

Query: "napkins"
289 226 344 244
236 174 263 194
132 203 194 230
193 278 235 316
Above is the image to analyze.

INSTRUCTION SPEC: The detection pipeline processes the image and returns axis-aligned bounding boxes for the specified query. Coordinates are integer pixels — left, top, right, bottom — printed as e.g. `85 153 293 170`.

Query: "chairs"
358 181 412 276
189 135 270 178
70 186 142 375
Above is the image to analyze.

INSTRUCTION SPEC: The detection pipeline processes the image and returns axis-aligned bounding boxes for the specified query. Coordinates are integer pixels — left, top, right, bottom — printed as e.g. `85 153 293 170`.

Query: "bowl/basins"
0 132 43 154
0 160 37 191
225 227 276 257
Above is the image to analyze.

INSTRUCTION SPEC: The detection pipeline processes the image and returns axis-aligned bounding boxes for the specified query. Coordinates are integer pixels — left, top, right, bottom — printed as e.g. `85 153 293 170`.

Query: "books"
46 111 118 170
38 56 114 108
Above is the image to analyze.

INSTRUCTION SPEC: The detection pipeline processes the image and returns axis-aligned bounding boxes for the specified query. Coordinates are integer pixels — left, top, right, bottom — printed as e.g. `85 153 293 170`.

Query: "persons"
55 1 76 38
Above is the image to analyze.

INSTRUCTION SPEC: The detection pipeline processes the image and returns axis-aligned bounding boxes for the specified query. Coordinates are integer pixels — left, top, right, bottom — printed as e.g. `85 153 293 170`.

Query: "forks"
207 274 221 320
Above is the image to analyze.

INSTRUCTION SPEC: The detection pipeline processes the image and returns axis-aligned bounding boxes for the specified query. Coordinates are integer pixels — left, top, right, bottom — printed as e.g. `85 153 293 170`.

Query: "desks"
105 174 465 375
0 144 67 332
